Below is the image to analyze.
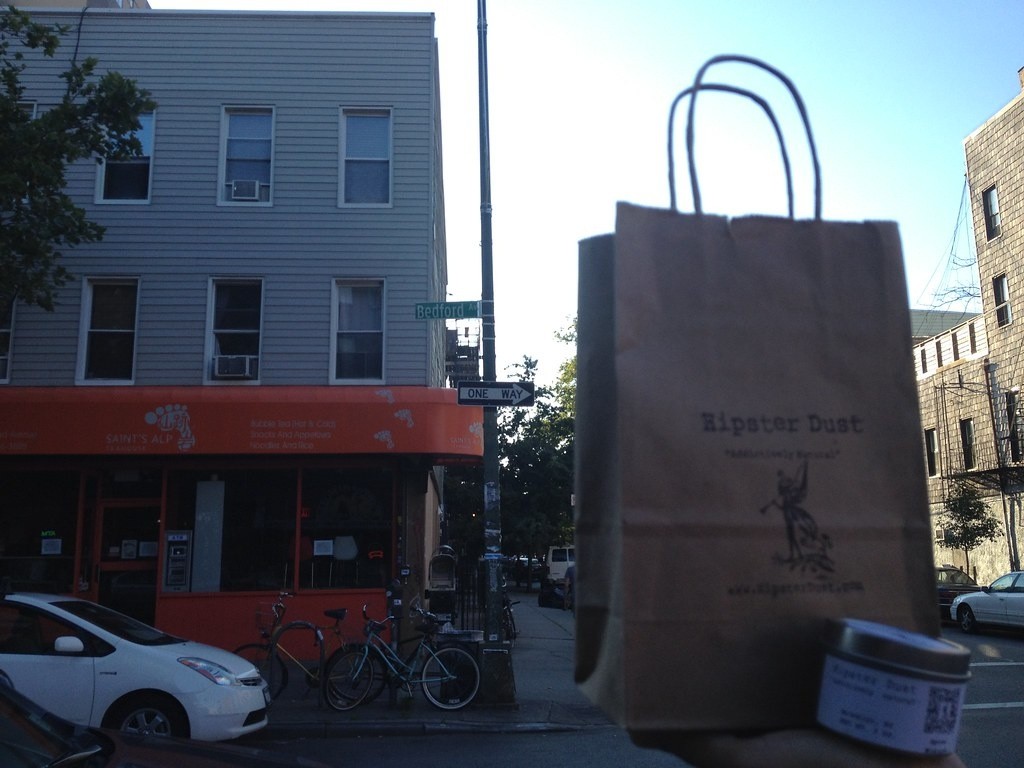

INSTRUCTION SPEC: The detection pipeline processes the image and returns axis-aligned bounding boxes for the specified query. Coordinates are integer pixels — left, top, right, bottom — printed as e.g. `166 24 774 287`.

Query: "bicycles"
231 592 484 713
502 576 522 648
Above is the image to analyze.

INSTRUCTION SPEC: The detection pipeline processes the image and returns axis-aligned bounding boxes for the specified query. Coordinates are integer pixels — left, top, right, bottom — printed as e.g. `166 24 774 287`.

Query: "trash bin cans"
430 629 485 701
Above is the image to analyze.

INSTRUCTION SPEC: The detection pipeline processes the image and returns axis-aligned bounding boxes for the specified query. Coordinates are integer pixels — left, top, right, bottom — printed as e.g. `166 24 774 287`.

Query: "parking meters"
424 545 460 695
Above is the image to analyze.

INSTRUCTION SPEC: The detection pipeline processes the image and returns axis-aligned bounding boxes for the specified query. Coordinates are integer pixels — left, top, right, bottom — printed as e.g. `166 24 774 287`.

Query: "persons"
563 566 576 619
514 559 525 587
628 714 967 768
540 561 550 595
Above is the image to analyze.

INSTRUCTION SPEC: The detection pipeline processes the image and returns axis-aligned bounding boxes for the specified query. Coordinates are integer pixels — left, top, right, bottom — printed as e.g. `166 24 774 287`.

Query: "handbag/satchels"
573 54 941 733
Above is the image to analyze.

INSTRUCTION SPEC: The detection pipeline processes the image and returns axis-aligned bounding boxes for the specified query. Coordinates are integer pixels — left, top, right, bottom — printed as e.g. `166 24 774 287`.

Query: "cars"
0 669 337 768
0 590 274 741
540 544 576 588
935 568 989 627
949 570 1024 634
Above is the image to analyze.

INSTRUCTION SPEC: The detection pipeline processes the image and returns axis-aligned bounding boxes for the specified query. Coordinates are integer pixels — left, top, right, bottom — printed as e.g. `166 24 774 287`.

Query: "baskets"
255 602 274 628
